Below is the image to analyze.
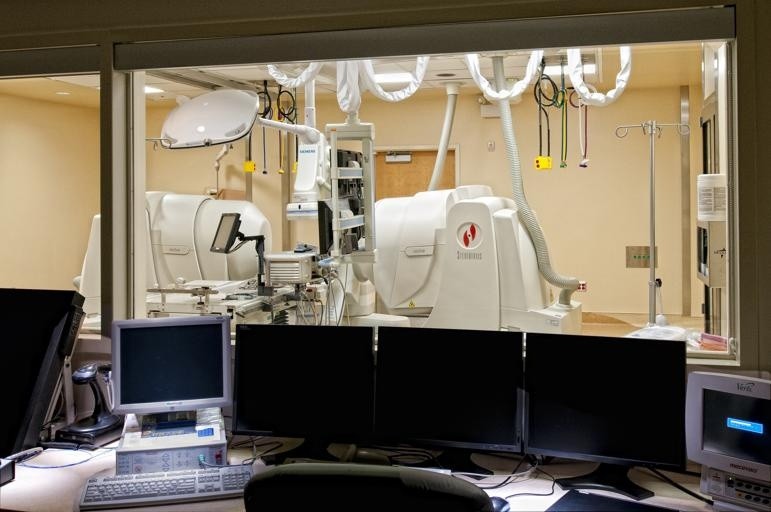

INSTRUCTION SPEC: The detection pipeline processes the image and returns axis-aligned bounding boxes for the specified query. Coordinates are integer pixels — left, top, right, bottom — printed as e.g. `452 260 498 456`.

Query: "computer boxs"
116 407 227 476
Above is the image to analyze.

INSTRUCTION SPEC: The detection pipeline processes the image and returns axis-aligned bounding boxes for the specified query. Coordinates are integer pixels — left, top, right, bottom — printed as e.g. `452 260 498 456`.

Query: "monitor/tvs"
210 213 241 253
378 326 524 480
685 369 771 482
231 323 377 467
110 314 231 438
524 333 687 502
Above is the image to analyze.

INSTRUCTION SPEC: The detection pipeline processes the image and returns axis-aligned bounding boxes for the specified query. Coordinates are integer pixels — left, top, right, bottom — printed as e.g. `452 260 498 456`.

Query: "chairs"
244 463 493 510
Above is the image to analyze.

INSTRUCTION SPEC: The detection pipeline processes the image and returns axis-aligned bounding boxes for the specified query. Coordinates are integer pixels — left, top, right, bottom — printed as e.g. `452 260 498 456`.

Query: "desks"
0 392 771 512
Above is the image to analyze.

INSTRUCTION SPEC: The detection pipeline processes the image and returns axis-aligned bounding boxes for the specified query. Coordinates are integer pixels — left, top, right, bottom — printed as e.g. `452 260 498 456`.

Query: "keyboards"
80 465 252 508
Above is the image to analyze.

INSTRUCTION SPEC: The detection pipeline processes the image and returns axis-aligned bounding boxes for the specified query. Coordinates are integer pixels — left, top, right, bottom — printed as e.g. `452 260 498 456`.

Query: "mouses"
489 496 510 512
